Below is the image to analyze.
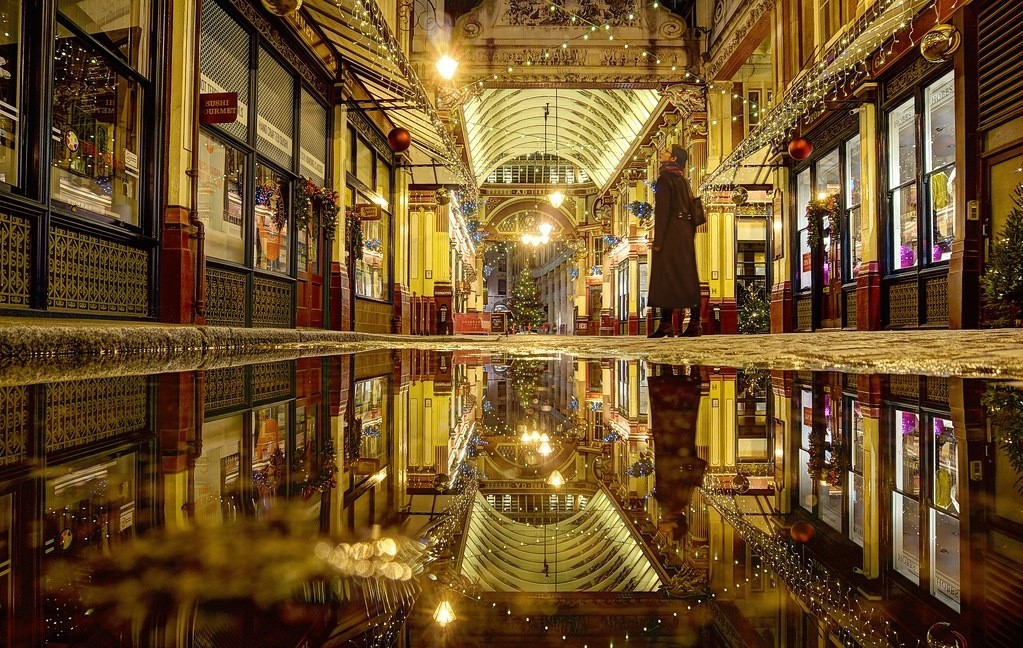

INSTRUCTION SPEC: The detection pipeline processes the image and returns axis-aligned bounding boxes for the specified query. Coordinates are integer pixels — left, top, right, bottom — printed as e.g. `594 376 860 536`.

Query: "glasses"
664 148 677 157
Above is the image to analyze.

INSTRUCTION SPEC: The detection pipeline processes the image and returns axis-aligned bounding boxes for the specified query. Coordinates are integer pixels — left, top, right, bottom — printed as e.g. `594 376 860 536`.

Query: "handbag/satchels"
689 196 707 226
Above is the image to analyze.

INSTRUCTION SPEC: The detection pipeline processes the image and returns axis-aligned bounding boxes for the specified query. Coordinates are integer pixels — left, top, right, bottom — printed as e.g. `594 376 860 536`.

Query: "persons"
648 365 706 539
646 144 702 338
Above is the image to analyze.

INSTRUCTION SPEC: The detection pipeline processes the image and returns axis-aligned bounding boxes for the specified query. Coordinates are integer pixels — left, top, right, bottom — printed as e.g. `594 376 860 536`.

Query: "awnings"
303 0 459 168
702 0 929 195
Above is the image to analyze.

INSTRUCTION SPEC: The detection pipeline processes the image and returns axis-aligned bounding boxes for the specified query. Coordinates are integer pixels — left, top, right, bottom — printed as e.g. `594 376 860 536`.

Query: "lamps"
518 89 566 247
521 431 565 595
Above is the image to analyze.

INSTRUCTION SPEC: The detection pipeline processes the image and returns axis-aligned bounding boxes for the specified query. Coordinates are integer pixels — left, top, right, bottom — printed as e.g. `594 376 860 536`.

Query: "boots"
678 318 700 337
647 323 674 338
